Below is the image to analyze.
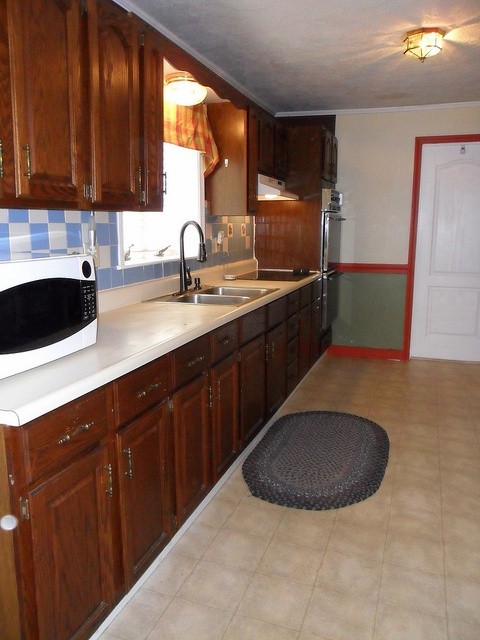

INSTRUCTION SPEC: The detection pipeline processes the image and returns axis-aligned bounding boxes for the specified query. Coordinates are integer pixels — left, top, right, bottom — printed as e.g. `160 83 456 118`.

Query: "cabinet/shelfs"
287 284 300 399
300 271 322 381
276 115 338 184
3 341 174 640
169 311 242 534
242 292 287 452
0 1 93 210
259 106 287 181
88 1 168 211
207 102 257 216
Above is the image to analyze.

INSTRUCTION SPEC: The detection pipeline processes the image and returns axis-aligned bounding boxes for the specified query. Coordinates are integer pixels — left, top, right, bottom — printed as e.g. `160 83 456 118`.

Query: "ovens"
321 187 346 332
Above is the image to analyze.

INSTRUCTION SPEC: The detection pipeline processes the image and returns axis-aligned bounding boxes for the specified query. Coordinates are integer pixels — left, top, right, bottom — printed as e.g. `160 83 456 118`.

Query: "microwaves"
0 253 97 382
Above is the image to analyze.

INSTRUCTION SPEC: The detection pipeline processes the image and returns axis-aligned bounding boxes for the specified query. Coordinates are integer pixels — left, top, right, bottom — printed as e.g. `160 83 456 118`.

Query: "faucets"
172 220 207 296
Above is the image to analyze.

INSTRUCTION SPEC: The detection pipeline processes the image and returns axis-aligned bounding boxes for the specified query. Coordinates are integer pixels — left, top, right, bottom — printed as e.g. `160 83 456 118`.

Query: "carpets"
243 411 389 510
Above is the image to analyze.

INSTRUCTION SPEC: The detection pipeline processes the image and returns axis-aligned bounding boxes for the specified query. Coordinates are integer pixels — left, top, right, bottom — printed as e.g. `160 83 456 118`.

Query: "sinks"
170 294 249 305
198 286 268 296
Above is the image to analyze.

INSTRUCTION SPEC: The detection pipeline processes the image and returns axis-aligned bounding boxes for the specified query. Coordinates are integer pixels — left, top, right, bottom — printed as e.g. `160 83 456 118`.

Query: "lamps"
401 27 445 63
167 75 209 109
